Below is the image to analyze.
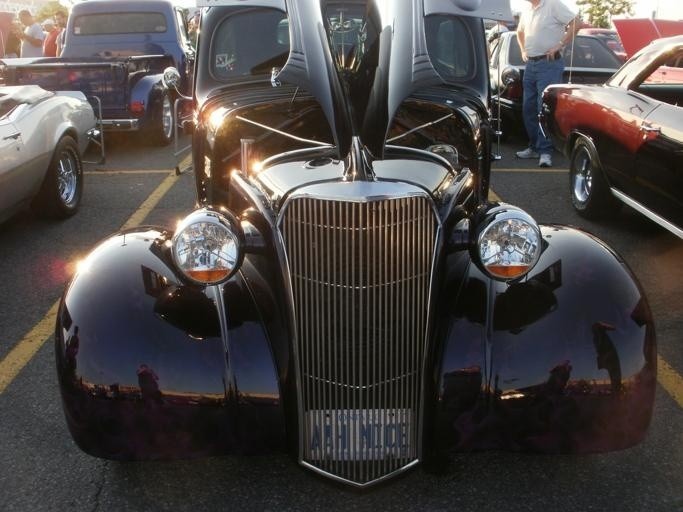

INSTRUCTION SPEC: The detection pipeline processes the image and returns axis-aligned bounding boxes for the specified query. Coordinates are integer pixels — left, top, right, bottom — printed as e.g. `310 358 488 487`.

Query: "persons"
488 21 508 43
0 11 66 59
516 0 582 168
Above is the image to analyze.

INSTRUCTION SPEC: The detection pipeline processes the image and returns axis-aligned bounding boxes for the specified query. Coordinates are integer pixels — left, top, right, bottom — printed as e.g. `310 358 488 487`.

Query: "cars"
487 32 625 140
0 1 197 146
578 27 628 64
55 0 682 490
0 84 105 223
539 35 682 241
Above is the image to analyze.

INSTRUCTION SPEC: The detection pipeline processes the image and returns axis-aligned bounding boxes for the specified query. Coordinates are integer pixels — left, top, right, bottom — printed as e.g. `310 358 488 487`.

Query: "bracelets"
559 41 566 49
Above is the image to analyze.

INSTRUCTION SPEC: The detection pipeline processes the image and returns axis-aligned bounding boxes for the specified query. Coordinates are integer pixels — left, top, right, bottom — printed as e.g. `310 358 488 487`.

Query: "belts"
528 50 559 62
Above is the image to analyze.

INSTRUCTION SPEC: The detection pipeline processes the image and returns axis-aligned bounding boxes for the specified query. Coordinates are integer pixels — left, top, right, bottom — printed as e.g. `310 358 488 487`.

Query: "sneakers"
538 153 552 167
516 148 540 158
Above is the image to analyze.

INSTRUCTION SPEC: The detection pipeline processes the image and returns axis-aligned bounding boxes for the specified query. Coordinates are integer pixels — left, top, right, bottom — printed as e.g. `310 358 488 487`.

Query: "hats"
41 19 55 25
